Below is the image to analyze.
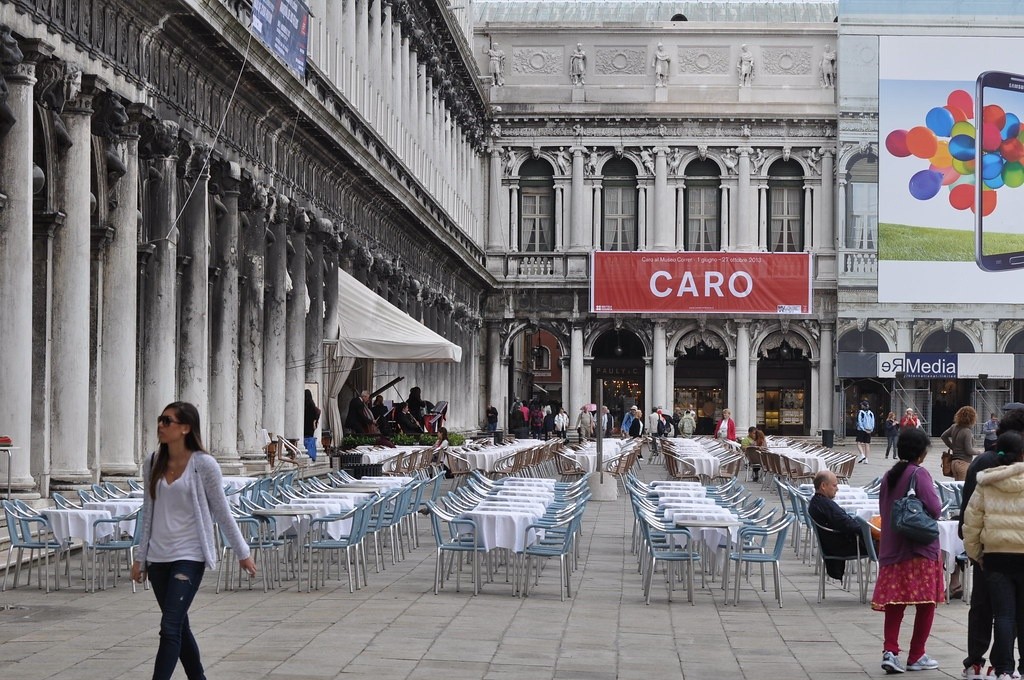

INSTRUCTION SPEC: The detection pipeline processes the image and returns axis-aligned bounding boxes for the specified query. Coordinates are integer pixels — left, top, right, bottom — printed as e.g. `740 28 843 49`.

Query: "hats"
630 406 637 411
860 401 869 407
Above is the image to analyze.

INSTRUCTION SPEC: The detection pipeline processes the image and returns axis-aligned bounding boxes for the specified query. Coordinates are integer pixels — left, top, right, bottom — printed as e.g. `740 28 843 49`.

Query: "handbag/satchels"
891 466 940 545
940 451 955 478
658 420 665 437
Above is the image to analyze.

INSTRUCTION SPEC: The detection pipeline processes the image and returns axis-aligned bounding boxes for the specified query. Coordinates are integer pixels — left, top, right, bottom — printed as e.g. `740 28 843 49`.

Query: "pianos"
368 376 405 407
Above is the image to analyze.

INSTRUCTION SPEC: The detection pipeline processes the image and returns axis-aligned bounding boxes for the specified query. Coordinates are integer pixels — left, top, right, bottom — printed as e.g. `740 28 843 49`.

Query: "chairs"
0 436 966 606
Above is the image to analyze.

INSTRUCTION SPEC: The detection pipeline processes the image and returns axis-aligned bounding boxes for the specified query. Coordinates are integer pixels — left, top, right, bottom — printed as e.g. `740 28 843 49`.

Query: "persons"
482 43 506 86
486 405 498 431
575 404 614 441
742 426 767 481
649 404 696 455
621 405 646 460
808 470 879 556
509 397 569 439
305 389 321 437
130 401 258 680
569 43 586 85
856 401 874 464
875 408 921 459
941 406 981 481
944 412 1024 680
818 44 835 86
345 387 425 434
714 409 735 441
737 44 755 88
651 44 670 87
432 427 448 462
870 427 945 673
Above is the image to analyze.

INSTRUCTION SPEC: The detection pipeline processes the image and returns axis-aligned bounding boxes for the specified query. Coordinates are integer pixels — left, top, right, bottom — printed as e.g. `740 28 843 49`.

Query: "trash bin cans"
822 429 834 448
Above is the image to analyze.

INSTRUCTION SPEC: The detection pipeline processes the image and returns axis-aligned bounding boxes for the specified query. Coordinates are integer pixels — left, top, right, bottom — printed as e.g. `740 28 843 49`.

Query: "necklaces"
168 458 184 475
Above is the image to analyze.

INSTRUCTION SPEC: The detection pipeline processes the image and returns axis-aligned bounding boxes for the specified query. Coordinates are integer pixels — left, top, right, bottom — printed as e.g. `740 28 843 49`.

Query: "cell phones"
973 71 1024 273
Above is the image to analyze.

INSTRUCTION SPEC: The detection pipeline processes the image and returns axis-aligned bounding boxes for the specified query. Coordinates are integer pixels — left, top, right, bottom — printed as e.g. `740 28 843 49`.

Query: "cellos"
348 383 381 434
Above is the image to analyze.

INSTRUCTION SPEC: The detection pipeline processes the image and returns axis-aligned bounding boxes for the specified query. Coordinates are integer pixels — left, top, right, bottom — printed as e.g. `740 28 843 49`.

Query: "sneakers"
983 666 997 680
996 673 1024 680
961 664 983 680
906 654 939 671
881 650 906 673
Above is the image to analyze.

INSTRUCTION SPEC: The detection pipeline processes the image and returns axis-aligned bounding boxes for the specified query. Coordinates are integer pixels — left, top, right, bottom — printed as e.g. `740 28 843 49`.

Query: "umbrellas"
580 404 596 411
1001 402 1024 410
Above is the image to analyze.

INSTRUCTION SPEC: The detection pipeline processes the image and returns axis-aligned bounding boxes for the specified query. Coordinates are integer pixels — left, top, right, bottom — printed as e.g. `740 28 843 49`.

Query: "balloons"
886 90 1024 217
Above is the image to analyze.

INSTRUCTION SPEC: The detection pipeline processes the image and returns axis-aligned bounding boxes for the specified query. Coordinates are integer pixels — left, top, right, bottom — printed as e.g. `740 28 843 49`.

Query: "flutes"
407 410 424 431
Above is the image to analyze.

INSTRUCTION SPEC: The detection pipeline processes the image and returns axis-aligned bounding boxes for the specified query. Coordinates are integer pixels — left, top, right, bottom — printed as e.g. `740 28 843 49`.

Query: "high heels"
944 585 963 600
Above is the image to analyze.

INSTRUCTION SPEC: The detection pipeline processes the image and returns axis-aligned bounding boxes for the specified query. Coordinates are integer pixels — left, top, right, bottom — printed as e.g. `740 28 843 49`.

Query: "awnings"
337 259 462 364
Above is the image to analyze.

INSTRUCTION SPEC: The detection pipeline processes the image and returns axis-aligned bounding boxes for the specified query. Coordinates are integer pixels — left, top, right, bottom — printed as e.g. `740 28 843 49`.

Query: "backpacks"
532 412 542 424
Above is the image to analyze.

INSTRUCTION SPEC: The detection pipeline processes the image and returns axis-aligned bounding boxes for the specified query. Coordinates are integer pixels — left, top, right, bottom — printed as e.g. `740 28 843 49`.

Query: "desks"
460 511 536 599
39 510 112 591
933 521 967 602
277 501 341 584
674 512 745 606
249 509 318 594
85 501 140 588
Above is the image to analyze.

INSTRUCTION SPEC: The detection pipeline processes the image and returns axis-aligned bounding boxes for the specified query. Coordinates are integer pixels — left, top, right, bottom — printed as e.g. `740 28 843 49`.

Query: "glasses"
157 415 184 427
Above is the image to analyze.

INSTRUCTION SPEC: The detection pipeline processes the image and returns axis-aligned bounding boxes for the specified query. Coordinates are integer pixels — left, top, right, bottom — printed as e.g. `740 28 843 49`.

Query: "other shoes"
863 458 868 464
857 454 865 464
